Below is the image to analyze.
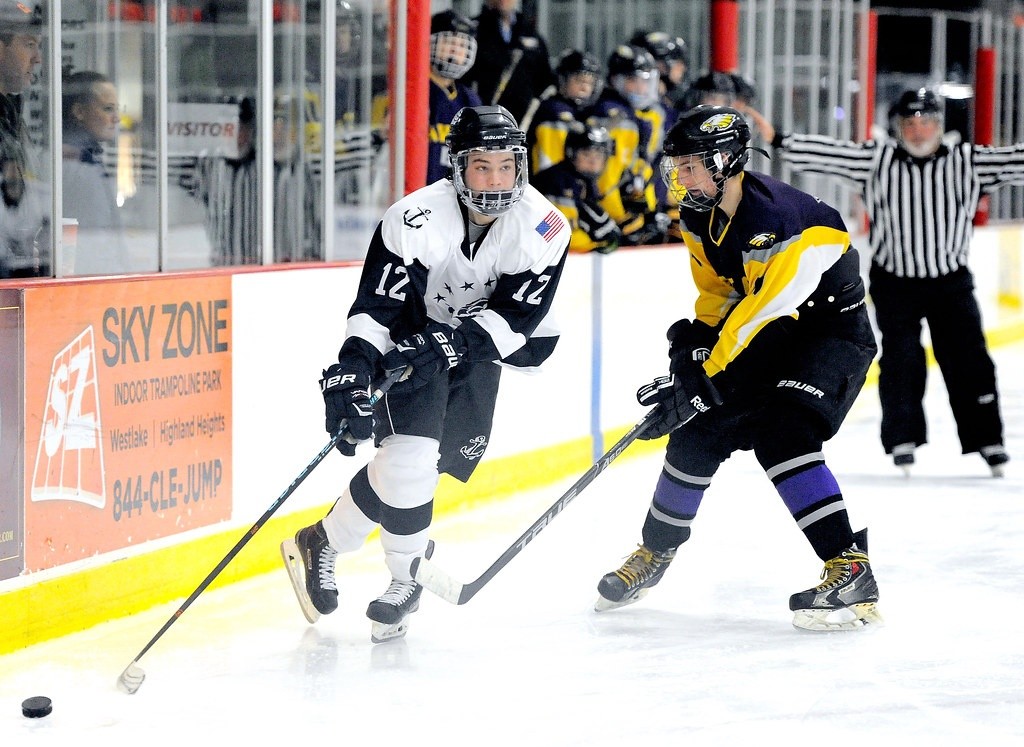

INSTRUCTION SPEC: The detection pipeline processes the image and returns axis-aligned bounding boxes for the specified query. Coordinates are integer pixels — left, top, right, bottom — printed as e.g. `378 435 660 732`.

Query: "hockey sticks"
120 371 404 695
411 409 664 607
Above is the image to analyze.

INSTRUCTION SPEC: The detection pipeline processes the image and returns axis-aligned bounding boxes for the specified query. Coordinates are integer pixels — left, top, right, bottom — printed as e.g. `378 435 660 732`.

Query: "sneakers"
979 443 1014 477
279 520 339 624
366 578 423 646
594 544 678 613
789 527 884 633
892 442 929 479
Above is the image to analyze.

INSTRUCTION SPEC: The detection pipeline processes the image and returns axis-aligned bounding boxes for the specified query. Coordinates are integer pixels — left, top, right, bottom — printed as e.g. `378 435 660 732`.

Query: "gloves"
381 321 468 397
319 364 374 456
635 361 722 440
666 318 720 374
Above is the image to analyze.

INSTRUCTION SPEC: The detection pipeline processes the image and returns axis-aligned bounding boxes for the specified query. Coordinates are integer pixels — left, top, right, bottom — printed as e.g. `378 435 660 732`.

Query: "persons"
281 105 573 643
1 1 129 274
183 0 759 253
594 103 880 631
62 93 386 265
742 87 1024 478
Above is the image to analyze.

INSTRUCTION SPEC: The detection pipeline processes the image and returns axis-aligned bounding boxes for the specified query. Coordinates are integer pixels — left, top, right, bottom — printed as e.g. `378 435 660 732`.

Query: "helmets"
609 45 658 105
899 90 941 115
430 10 477 79
216 92 321 160
659 105 751 210
631 30 756 101
551 48 606 105
563 124 611 179
442 104 529 217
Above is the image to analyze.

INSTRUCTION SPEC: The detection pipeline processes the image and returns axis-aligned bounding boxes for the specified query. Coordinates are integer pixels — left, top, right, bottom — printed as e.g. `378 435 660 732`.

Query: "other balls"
21 696 52 718
